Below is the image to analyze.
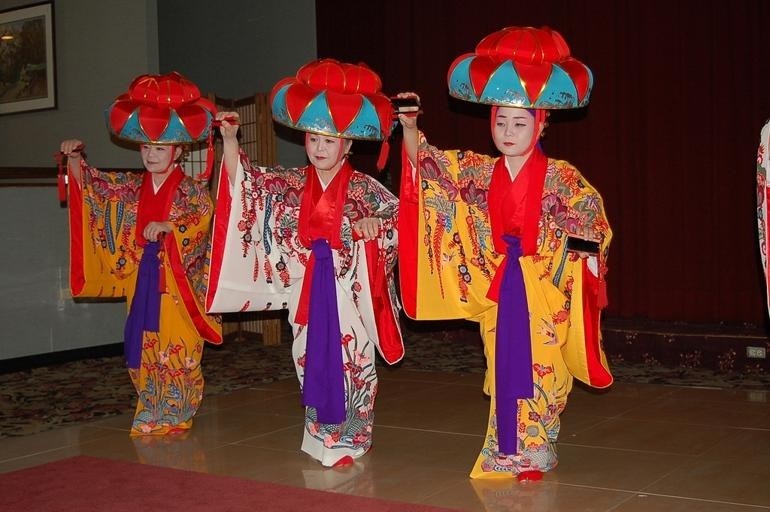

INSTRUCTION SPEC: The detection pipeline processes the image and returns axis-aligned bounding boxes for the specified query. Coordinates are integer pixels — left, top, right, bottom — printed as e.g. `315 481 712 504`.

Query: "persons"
755 121 770 319
397 25 615 484
214 56 406 470
59 70 224 438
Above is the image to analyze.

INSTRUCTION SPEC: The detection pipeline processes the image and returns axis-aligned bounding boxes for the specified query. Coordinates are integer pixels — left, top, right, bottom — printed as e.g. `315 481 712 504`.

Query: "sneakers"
332 455 355 469
517 471 545 484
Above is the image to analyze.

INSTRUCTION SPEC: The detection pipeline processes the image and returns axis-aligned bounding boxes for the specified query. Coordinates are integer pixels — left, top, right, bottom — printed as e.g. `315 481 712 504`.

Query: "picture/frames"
0 2 58 117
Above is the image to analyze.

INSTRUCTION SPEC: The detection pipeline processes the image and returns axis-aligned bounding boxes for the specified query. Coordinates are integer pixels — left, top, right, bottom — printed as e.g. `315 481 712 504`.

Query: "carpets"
2 454 465 512
